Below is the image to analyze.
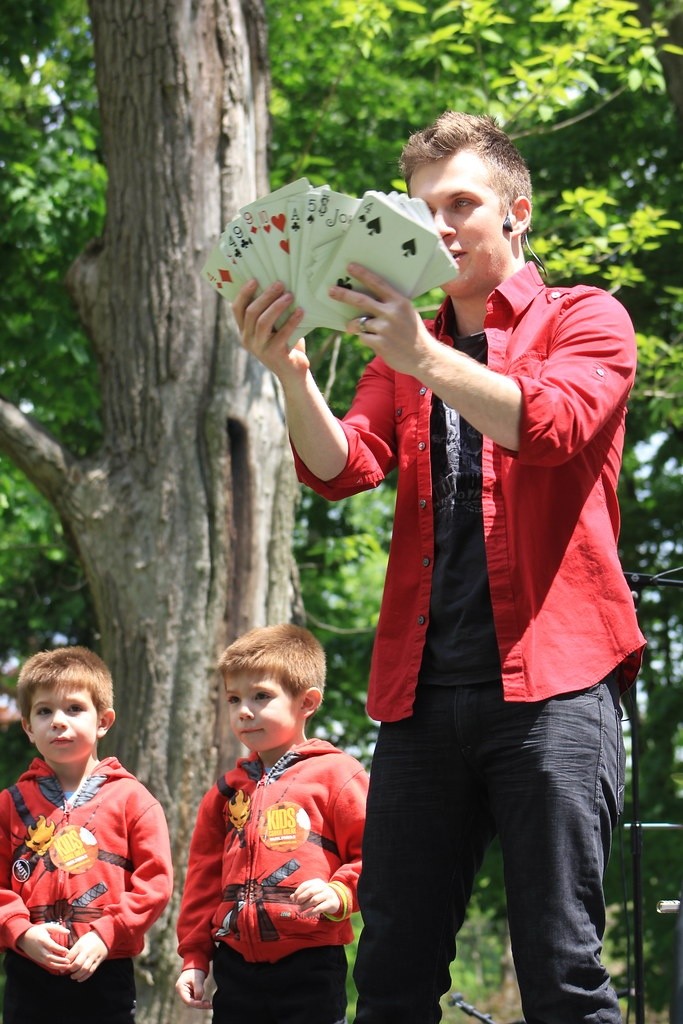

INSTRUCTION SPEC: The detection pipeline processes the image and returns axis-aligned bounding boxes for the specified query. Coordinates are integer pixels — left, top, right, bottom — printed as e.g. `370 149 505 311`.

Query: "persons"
231 113 641 1022
0 647 171 1024
176 625 370 1023
0 657 20 736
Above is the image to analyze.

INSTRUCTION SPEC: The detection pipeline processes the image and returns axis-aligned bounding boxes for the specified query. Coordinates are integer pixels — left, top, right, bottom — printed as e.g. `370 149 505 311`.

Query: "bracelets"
322 880 352 922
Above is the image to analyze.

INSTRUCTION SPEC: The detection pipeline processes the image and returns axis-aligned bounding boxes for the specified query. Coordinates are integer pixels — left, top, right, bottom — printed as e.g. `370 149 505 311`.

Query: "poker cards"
198 175 460 353
